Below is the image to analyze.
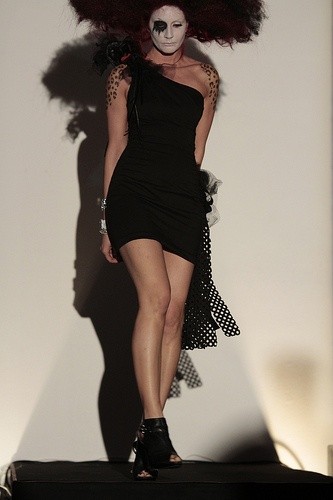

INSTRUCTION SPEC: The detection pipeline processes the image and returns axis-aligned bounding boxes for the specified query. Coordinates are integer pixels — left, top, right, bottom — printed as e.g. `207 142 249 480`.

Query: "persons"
99 2 220 481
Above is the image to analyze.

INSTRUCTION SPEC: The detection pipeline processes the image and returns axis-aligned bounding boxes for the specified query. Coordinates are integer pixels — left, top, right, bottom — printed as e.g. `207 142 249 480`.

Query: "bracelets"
99 198 106 234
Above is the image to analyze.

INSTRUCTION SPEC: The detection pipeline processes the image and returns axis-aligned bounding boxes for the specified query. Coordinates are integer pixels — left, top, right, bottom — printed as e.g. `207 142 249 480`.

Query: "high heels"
137 418 182 468
131 439 158 481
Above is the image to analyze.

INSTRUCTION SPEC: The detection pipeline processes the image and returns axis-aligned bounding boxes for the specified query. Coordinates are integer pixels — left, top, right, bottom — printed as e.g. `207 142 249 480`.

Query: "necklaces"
161 51 183 68
